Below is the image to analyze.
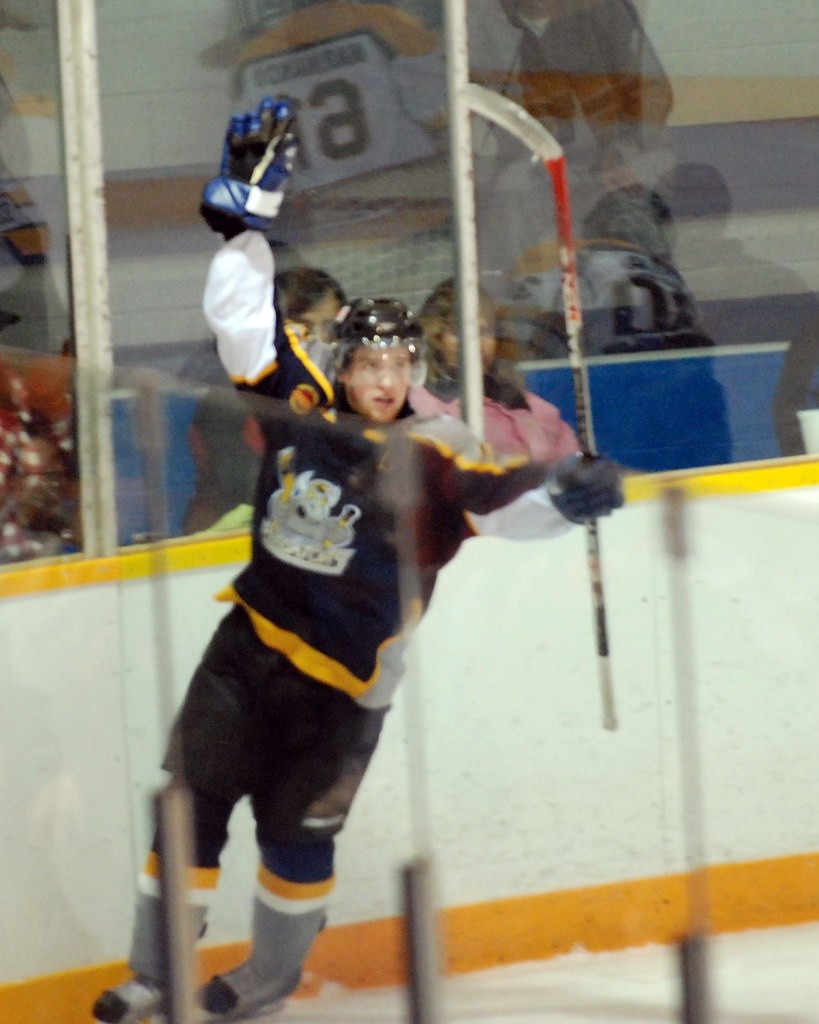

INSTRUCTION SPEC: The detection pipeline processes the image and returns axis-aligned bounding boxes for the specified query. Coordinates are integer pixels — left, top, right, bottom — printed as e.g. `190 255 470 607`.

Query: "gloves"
553 446 625 519
199 97 299 230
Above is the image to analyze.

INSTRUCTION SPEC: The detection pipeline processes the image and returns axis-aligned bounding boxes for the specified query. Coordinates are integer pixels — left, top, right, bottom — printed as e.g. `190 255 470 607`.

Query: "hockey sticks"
463 84 619 731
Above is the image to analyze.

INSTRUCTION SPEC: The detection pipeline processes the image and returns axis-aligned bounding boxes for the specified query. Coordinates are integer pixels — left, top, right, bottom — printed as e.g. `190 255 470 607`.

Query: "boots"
197 899 330 1022
92 883 212 1022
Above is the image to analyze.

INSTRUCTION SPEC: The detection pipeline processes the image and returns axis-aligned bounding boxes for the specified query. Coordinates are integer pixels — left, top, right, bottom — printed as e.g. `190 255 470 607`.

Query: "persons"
480 0 674 171
496 181 734 476
413 275 582 467
171 264 350 535
215 0 502 242
89 94 624 1024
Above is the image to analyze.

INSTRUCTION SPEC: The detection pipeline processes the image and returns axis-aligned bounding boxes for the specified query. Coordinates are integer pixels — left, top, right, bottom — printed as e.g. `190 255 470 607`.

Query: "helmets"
577 184 681 262
332 296 423 359
